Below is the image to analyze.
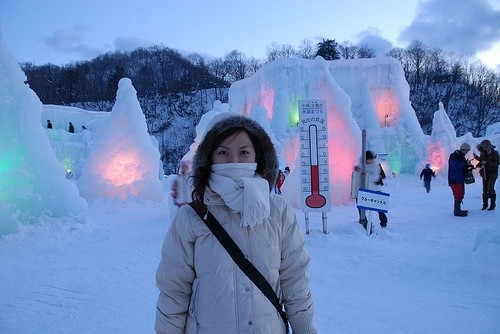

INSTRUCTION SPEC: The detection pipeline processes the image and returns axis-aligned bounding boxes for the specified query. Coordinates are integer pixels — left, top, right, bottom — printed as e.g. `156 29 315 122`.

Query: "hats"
425 164 429 168
366 151 376 159
460 143 471 150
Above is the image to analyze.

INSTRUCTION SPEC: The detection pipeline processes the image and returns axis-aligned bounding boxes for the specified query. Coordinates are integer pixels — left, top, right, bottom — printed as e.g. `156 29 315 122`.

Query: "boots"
488 194 496 210
482 193 488 210
454 201 466 216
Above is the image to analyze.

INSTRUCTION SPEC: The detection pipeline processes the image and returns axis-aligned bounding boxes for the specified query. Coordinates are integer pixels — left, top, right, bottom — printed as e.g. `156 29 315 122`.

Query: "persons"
355 150 387 234
82 125 87 130
448 143 471 217
47 120 52 129
420 163 436 193
154 115 317 334
69 122 74 133
172 161 194 208
472 140 500 210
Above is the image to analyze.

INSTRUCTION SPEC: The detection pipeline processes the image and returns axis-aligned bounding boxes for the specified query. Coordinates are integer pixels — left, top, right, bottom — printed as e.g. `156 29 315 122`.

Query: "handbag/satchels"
465 169 475 184
